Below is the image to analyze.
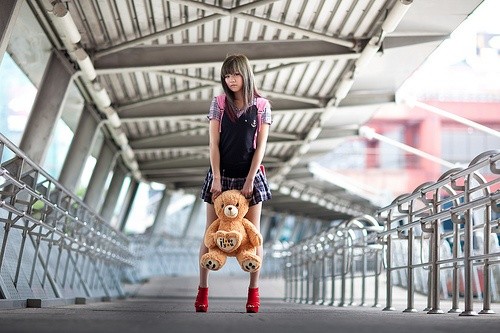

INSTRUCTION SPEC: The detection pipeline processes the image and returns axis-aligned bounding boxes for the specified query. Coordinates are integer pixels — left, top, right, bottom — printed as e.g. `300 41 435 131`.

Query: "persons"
193 56 273 317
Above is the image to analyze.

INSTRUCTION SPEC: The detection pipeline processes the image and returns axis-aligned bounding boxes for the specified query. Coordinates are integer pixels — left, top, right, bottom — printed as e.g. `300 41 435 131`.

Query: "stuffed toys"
200 189 263 273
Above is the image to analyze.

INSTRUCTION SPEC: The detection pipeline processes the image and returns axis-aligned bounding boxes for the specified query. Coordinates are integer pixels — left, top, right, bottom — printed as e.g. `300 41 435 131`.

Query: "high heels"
195 286 209 312
246 288 260 312
